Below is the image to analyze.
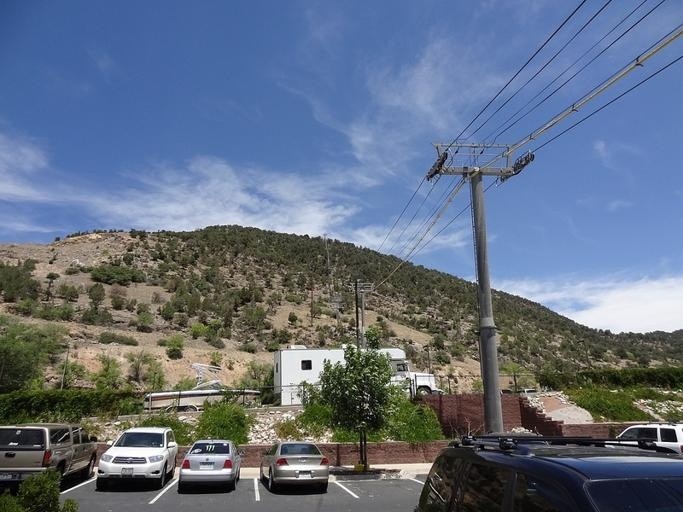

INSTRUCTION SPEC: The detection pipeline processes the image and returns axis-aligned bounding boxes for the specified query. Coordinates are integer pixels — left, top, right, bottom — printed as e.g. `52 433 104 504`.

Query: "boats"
140 380 260 415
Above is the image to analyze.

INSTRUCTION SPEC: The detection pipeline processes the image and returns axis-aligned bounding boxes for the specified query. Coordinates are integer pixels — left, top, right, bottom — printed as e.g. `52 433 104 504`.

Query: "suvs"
617 425 681 456
93 426 178 490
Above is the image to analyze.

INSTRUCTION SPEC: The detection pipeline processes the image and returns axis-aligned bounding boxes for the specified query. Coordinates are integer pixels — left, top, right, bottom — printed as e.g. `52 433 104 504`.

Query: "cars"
257 440 330 494
175 437 245 493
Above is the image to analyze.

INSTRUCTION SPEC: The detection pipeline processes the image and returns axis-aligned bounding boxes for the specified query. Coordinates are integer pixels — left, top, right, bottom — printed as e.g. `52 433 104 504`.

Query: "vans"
0 420 99 490
413 429 681 512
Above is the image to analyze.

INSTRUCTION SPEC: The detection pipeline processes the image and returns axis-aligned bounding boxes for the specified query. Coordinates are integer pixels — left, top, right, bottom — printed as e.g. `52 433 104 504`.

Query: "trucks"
268 341 435 403
